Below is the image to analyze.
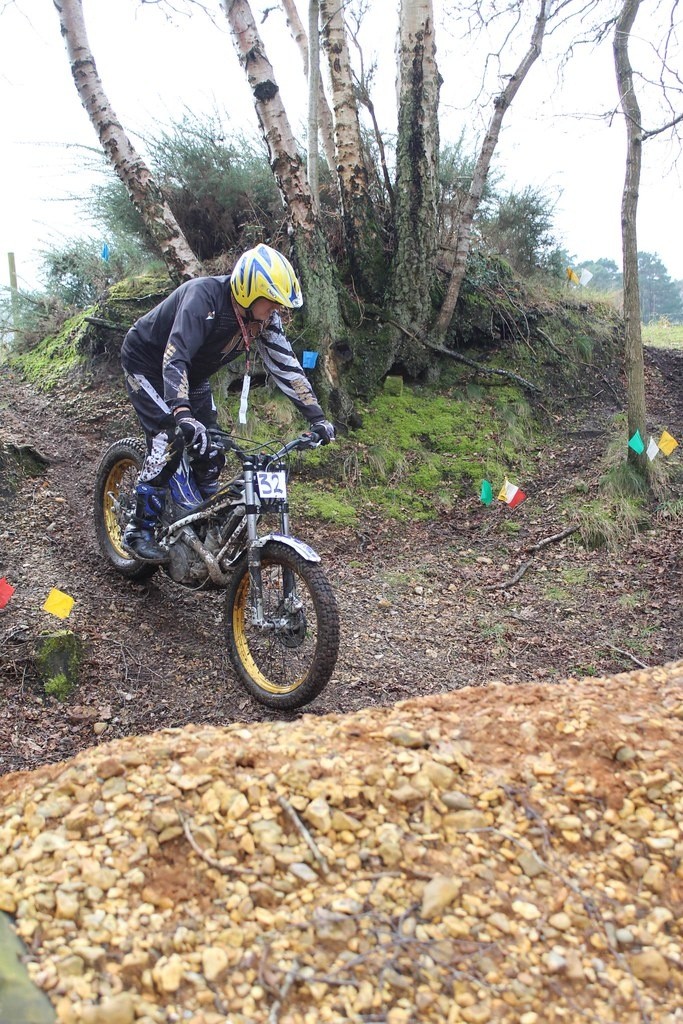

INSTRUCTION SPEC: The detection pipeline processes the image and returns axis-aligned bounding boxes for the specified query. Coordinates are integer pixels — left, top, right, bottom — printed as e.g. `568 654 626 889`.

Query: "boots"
120 480 170 563
199 483 220 501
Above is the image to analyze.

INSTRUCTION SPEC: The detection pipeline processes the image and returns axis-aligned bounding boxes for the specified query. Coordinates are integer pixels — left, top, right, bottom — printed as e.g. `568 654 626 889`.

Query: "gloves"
175 410 211 456
310 419 337 446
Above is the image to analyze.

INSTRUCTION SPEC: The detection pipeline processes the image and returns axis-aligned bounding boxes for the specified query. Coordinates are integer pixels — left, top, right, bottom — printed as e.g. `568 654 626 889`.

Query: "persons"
120 242 335 565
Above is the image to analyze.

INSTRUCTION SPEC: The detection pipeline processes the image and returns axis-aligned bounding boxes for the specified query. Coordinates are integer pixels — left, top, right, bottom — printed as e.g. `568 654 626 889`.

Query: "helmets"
230 244 303 309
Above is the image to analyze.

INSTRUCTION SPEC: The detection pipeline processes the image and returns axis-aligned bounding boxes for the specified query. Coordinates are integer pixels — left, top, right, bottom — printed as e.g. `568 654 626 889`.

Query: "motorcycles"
95 429 341 710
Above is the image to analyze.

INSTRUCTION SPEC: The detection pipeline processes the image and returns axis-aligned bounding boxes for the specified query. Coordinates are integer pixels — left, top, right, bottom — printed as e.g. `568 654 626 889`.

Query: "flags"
627 427 678 462
0 577 16 609
497 478 527 508
101 242 110 261
480 480 493 507
43 588 74 620
302 351 318 369
